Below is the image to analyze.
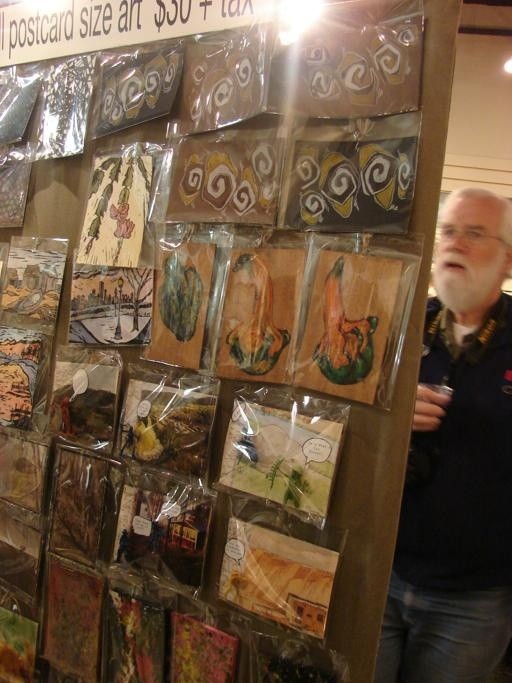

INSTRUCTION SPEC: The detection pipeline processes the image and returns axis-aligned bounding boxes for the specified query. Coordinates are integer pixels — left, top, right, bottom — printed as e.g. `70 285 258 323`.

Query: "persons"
374 183 511 681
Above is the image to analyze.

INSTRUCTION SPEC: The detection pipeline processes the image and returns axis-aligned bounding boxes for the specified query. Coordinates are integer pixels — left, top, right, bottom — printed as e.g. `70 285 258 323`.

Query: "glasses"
440 229 506 246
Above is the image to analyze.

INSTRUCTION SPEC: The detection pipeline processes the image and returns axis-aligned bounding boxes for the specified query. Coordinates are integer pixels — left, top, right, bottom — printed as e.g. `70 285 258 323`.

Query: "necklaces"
419 292 504 388
